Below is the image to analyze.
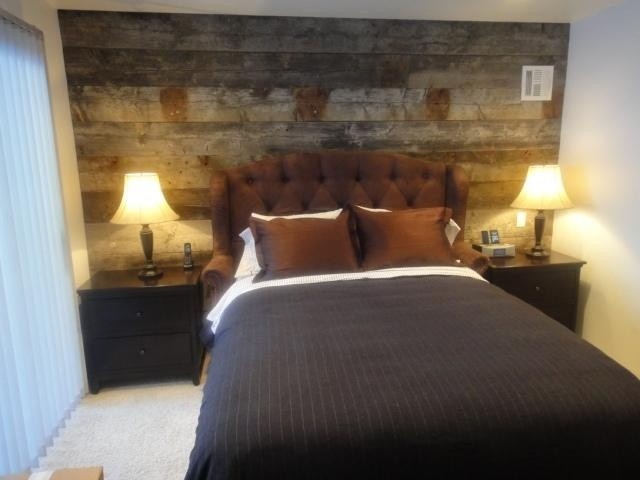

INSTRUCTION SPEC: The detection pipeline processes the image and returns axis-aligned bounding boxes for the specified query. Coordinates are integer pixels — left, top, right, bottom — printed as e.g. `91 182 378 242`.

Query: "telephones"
183 243 193 271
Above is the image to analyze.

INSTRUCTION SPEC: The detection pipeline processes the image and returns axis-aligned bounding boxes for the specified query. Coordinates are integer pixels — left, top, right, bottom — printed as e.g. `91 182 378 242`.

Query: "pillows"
248 208 359 283
348 203 456 271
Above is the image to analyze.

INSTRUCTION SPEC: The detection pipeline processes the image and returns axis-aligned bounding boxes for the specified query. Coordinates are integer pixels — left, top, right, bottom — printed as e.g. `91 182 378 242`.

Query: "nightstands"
488 247 587 334
76 268 206 395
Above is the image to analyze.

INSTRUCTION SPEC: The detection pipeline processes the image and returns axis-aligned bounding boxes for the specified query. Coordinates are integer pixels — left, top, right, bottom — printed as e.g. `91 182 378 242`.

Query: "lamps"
510 164 575 259
108 172 181 280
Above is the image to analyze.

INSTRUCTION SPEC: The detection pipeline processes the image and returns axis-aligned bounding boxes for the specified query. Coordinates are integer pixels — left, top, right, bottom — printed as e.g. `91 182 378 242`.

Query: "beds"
184 150 640 480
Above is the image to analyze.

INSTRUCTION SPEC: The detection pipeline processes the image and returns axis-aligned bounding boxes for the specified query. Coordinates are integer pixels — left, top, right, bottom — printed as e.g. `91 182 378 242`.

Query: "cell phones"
490 229 499 243
481 231 489 244
